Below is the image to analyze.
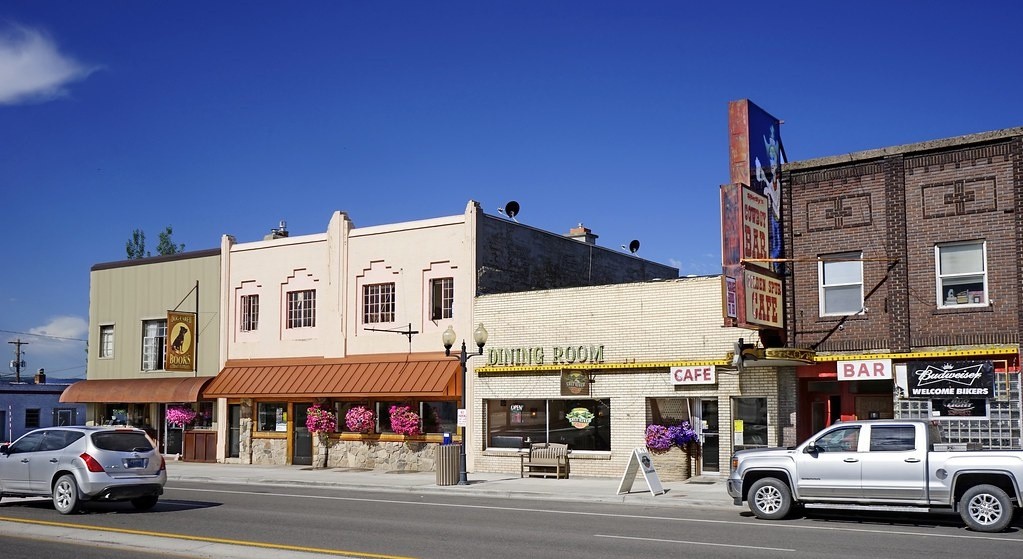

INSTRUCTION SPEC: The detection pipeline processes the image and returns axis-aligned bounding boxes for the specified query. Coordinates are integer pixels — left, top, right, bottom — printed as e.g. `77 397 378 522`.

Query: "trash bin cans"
436 443 460 487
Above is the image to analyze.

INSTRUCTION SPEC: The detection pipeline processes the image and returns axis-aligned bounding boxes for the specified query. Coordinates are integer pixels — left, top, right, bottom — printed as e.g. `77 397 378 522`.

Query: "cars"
0 425 168 516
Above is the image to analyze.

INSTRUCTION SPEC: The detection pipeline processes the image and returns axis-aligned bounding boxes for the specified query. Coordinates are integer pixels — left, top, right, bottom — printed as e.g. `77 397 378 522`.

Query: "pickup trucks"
727 418 1023 533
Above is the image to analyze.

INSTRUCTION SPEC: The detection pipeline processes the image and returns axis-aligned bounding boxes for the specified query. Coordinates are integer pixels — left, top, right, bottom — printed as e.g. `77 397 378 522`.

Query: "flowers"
345 404 376 433
305 401 337 438
165 406 197 427
645 421 698 453
389 404 421 437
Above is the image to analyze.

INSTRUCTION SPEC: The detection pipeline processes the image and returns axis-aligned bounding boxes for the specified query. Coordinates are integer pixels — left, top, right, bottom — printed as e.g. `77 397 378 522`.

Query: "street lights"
441 322 488 485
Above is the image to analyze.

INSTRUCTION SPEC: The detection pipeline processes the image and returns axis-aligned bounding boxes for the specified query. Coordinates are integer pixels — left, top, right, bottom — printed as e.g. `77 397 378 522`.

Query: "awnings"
58 378 216 403
201 352 463 400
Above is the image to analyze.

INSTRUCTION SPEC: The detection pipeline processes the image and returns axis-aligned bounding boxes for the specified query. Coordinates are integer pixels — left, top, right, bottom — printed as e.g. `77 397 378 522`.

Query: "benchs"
518 443 571 480
